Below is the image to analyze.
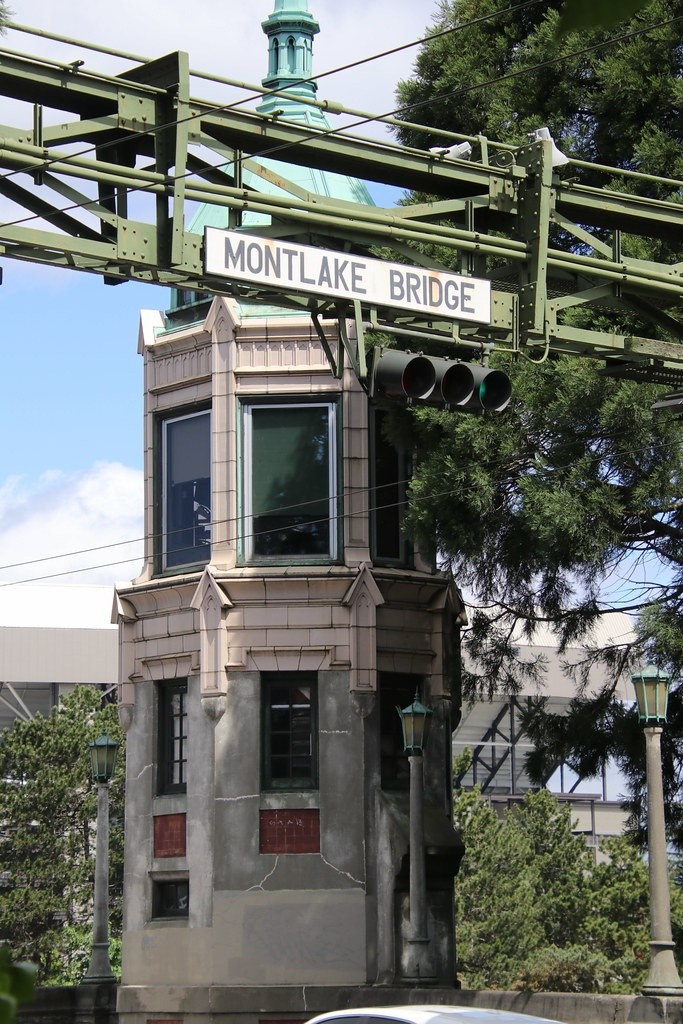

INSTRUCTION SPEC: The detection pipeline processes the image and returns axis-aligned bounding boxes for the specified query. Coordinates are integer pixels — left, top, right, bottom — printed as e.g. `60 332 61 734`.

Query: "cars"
303 1006 569 1024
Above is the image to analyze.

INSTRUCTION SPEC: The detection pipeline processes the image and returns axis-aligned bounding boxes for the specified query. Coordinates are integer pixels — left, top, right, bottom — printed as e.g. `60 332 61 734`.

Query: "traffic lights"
370 344 513 416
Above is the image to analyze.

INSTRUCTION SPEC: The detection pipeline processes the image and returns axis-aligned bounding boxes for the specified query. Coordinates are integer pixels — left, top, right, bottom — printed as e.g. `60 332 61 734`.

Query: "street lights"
84 734 124 985
395 692 440 985
631 663 683 995
